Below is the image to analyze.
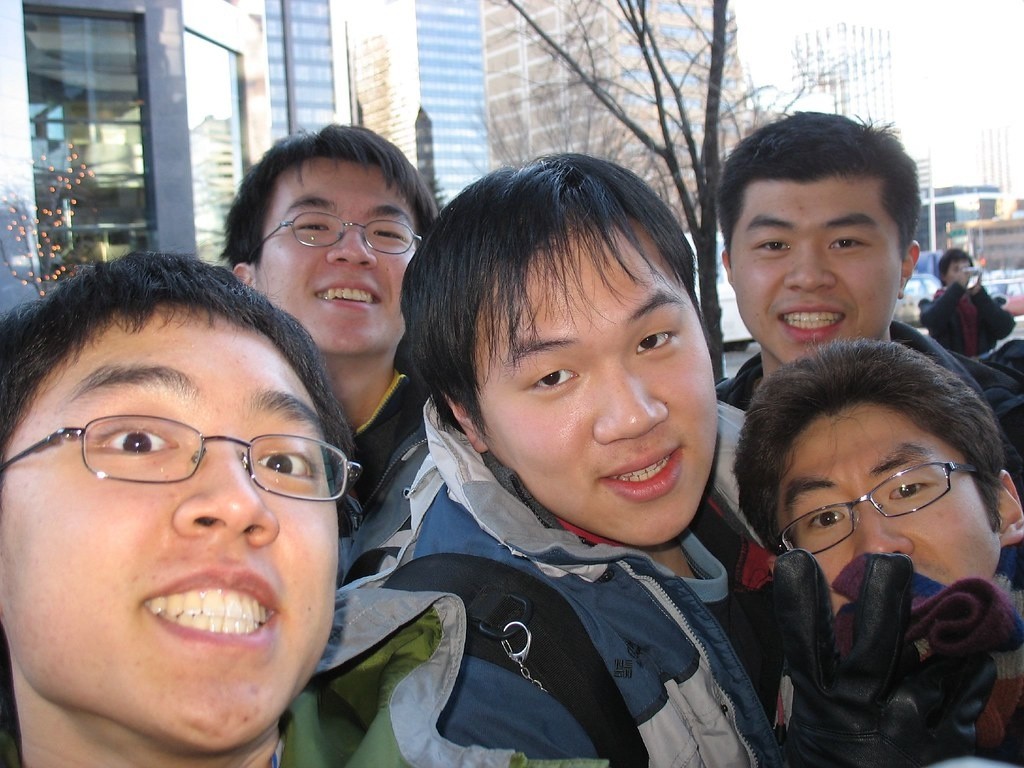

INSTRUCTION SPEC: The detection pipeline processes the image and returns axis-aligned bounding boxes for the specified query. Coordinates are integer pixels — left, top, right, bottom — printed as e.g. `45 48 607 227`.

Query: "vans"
684 232 755 352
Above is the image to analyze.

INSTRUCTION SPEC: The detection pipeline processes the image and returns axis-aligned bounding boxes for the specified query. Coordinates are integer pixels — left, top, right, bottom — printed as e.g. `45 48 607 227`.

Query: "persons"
733 335 1023 768
361 152 802 767
711 111 1023 451
0 253 361 768
221 123 436 501
918 247 1016 358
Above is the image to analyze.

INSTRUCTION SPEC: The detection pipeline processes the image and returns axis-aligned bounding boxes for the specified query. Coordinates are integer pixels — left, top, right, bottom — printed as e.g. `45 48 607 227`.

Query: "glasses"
771 461 978 555
0 415 362 502
248 211 422 254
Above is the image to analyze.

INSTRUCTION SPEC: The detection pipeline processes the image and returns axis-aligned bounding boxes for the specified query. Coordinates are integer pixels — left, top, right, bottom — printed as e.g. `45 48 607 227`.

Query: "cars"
891 274 945 327
980 279 1024 320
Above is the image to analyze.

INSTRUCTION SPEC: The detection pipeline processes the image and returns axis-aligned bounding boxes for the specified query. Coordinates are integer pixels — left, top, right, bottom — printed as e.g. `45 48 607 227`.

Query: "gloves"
767 551 998 768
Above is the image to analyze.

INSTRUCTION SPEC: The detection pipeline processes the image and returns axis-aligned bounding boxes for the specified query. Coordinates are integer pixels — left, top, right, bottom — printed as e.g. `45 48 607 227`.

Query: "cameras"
963 267 979 276
